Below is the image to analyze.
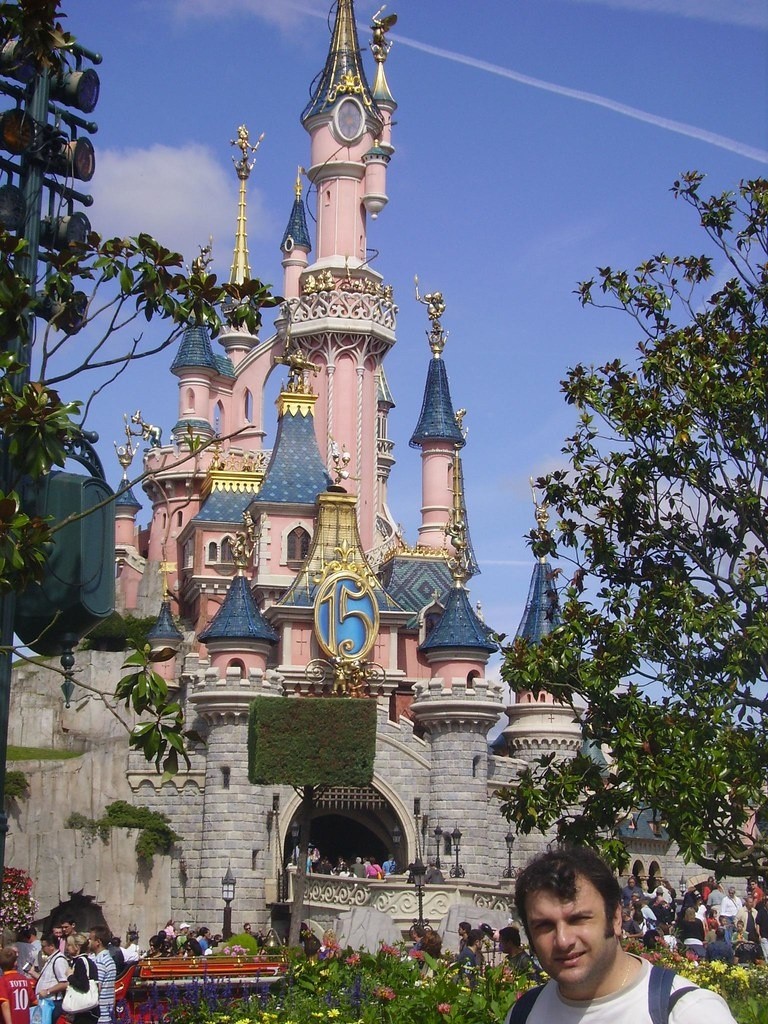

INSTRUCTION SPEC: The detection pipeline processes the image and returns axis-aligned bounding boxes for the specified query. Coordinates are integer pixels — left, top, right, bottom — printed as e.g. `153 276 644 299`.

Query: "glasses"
186 948 192 952
246 928 251 930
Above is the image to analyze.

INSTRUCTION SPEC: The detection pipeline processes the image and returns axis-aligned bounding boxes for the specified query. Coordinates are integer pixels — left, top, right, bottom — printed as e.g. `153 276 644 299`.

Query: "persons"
503 849 737 1024
412 922 443 958
142 919 222 958
0 918 140 1024
620 877 768 966
458 922 483 964
457 929 485 980
499 927 533 968
293 845 397 880
243 923 262 947
405 863 445 884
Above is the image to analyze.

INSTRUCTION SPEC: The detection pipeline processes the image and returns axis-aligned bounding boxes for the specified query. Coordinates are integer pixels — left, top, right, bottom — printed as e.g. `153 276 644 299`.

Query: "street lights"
1 1 112 907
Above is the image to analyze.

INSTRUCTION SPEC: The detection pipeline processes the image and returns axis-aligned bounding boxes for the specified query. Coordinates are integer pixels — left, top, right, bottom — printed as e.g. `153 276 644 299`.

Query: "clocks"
284 237 295 253
328 96 367 146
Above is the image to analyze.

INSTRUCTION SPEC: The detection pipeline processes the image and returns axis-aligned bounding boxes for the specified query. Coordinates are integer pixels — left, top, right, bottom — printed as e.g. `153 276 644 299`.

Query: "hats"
656 887 664 893
180 923 191 930
631 892 639 897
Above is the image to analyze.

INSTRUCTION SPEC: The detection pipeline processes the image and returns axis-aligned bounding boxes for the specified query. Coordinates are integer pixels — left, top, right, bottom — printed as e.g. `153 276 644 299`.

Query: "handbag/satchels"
377 871 381 880
29 996 55 1024
61 957 100 1013
